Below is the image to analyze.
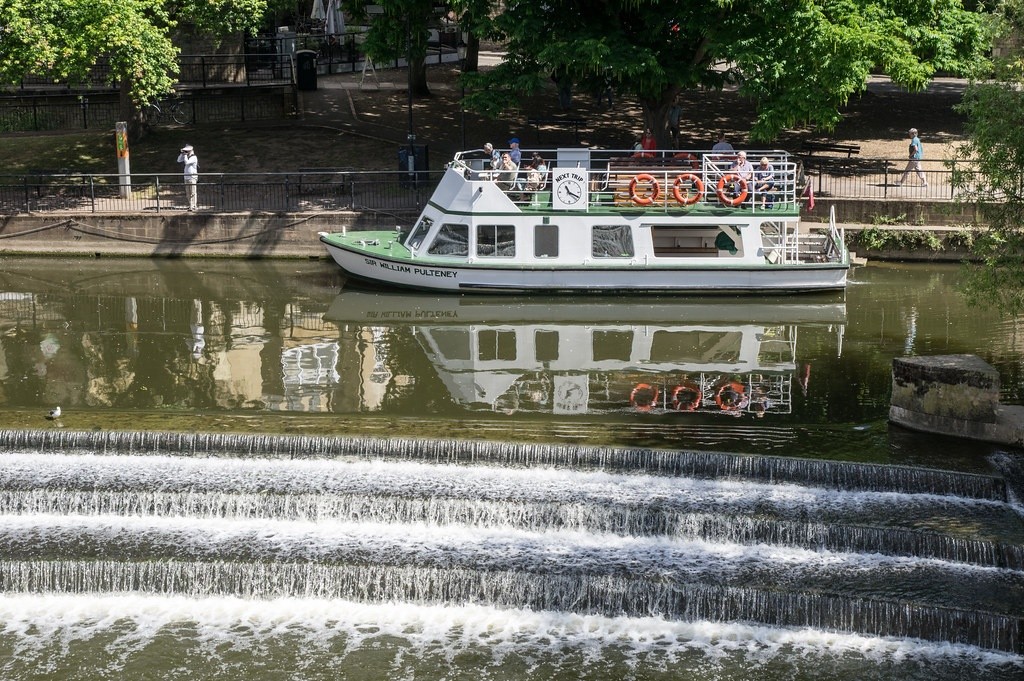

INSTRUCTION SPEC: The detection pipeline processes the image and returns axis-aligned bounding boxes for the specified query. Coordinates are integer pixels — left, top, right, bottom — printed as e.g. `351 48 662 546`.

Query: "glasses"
502 157 510 158
646 132 653 135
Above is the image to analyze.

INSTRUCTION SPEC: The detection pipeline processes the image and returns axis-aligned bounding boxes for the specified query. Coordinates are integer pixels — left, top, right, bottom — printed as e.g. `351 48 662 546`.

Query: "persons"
711 132 735 155
754 156 775 211
667 94 684 144
641 127 656 157
632 134 643 150
484 142 503 169
177 144 199 211
730 150 754 209
894 128 928 187
495 152 518 191
516 152 546 201
508 138 521 164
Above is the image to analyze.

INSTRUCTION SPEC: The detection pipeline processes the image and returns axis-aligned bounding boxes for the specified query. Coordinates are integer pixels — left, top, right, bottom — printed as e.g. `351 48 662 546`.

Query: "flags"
805 179 815 213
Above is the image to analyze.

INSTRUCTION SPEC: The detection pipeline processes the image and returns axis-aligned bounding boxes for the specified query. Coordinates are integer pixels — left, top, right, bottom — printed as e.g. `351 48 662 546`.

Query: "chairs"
507 161 521 190
524 161 550 206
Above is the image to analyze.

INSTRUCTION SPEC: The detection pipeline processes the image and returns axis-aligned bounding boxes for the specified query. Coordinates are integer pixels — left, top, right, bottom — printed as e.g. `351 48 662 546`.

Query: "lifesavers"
628 173 660 206
717 173 748 206
711 153 740 178
671 383 702 410
670 153 699 176
630 153 654 159
673 173 704 205
715 381 744 410
629 382 658 412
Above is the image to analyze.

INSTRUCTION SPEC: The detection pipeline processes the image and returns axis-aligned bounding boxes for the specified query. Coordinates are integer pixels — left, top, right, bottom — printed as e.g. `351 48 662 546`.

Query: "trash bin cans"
297 50 317 91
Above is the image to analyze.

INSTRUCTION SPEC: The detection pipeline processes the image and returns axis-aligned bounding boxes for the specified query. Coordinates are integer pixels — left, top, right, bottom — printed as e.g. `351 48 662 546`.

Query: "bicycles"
144 94 195 125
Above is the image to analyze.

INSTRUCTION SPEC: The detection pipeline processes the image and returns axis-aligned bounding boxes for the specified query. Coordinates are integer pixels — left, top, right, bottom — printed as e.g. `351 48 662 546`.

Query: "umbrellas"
310 0 325 20
324 0 345 46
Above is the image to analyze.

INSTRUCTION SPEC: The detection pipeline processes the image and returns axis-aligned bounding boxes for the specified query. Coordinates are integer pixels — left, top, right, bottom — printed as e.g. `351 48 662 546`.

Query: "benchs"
729 187 776 209
803 141 861 171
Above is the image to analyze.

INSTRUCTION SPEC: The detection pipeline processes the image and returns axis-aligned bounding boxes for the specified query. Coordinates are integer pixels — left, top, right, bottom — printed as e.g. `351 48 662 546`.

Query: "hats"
507 138 520 144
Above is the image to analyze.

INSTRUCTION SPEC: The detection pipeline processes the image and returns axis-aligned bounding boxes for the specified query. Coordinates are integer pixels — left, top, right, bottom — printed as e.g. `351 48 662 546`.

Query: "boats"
323 277 847 419
316 148 850 295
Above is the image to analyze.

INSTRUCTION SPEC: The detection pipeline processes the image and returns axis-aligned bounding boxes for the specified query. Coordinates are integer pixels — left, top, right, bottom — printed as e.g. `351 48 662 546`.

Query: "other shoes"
740 204 747 209
894 181 903 185
920 183 928 187
735 205 739 208
761 205 765 210
